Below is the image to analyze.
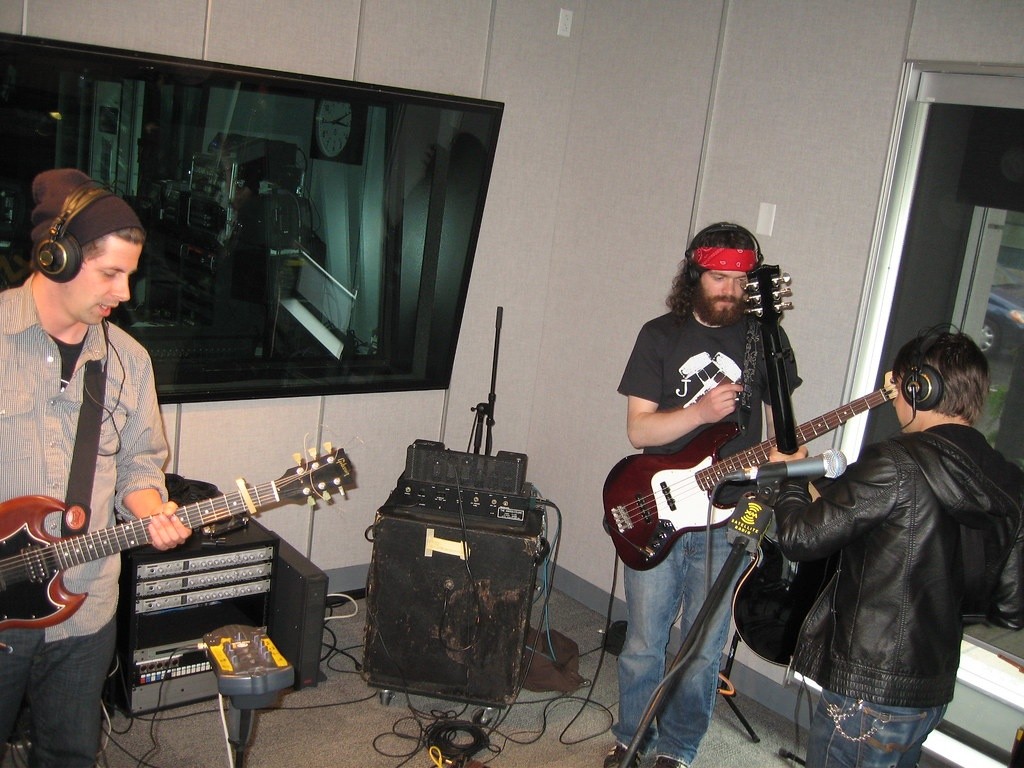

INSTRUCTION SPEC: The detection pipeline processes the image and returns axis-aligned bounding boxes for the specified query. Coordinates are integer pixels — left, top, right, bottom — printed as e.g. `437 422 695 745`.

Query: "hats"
31 169 144 271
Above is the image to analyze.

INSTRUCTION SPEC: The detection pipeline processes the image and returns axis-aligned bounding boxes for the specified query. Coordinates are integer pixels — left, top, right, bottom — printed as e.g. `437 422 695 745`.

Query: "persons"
770 333 1024 767
604 222 803 768
0 168 193 768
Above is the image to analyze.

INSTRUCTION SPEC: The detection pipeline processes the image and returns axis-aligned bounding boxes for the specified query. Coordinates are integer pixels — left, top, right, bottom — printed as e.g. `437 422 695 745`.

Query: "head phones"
32 180 125 284
901 322 964 411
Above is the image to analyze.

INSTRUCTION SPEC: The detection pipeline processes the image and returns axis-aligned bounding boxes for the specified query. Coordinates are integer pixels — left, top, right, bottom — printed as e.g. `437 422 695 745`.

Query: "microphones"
723 448 847 482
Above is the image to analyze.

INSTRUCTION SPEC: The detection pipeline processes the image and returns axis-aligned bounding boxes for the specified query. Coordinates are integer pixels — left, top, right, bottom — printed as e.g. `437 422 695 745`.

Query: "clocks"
308 101 368 165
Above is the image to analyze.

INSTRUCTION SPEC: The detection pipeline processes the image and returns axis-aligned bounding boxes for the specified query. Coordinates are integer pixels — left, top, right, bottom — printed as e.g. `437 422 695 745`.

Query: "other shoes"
652 755 687 768
604 743 641 768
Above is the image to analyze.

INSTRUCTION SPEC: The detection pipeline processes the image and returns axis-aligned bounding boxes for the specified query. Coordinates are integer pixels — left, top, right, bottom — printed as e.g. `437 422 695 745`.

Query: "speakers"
362 489 545 707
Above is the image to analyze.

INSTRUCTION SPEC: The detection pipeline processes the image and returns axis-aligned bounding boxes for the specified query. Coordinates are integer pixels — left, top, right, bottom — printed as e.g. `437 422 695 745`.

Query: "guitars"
731 254 844 668
0 437 358 627
599 372 901 570
